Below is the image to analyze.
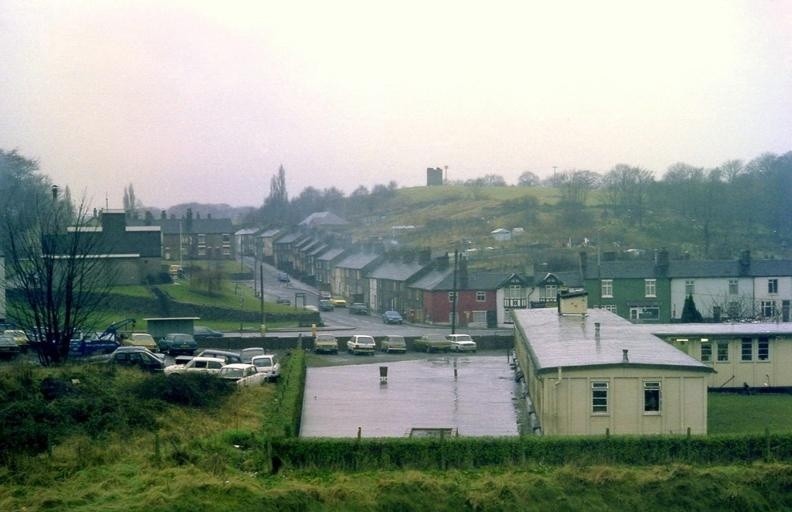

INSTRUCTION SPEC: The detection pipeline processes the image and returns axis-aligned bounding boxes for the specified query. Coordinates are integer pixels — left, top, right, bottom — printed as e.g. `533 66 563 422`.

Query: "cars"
318 290 331 299
413 332 451 353
329 296 347 309
0 324 282 392
276 296 292 306
319 299 333 312
278 272 290 283
346 333 377 355
312 333 339 355
346 301 369 316
381 310 403 325
304 304 319 316
380 334 407 355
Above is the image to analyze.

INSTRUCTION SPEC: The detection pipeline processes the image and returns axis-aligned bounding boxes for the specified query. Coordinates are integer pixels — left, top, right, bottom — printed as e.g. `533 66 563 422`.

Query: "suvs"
447 333 478 354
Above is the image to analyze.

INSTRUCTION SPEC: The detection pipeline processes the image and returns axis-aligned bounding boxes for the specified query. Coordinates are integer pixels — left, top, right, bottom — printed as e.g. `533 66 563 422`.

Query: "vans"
169 264 185 276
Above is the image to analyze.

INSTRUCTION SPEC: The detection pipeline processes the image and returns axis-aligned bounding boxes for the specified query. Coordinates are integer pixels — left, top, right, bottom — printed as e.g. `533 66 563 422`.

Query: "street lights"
595 225 609 309
